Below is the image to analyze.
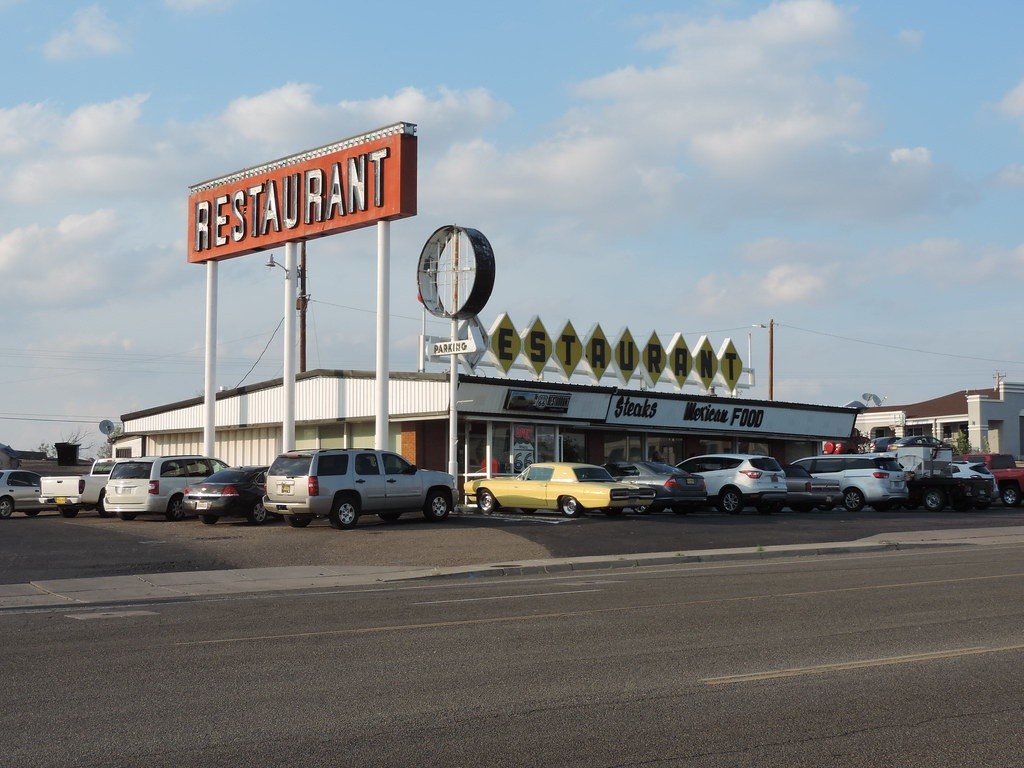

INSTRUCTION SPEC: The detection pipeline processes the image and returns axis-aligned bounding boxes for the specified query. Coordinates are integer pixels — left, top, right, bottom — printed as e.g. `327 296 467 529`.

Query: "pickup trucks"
952 450 1024 508
37 456 136 518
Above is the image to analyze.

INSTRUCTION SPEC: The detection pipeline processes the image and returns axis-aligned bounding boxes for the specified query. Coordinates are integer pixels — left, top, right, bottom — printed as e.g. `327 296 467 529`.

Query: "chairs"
166 466 177 476
359 458 374 472
190 464 200 474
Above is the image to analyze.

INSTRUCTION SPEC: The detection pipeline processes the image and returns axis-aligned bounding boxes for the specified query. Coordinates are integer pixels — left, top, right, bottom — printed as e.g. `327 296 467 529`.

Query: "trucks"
822 426 995 513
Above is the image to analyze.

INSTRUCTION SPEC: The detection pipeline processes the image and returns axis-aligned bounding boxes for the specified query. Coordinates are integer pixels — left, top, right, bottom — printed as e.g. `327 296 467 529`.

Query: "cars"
886 435 953 454
463 461 657 519
762 462 844 514
581 460 708 515
0 469 59 520
868 436 908 453
183 462 327 526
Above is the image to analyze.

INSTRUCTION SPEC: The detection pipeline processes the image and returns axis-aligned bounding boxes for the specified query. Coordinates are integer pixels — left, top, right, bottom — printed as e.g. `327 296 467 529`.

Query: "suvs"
939 459 1000 512
101 453 230 523
662 452 788 516
261 448 460 531
789 453 910 513
